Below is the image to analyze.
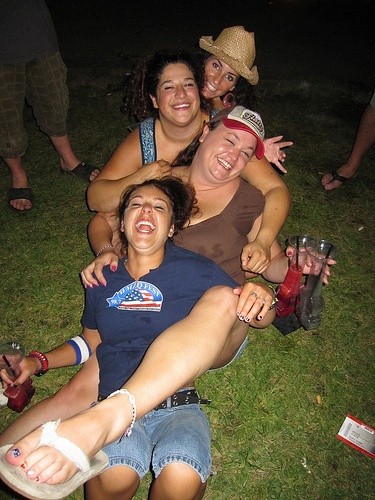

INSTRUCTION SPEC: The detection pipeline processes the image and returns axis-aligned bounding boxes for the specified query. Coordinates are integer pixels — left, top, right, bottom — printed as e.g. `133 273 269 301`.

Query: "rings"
262 297 265 303
251 292 257 297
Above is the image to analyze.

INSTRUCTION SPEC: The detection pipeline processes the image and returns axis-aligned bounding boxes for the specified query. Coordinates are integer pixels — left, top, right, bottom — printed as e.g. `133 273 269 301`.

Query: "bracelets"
29 351 48 377
268 285 278 310
97 245 112 256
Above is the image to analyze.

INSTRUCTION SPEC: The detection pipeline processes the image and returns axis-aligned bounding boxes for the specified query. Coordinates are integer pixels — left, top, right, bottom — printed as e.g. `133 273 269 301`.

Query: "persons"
87 49 293 270
0 175 276 500
0 0 101 212
0 106 337 500
199 26 294 174
320 92 375 192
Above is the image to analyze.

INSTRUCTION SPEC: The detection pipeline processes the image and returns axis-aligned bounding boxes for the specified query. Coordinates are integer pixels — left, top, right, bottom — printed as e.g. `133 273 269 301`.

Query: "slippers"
8 176 35 213
321 168 360 190
66 161 100 184
0 420 112 498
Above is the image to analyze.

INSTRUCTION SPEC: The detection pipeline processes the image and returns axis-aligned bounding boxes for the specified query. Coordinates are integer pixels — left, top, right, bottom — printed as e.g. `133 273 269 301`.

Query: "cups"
297 239 336 325
0 343 36 412
274 235 313 318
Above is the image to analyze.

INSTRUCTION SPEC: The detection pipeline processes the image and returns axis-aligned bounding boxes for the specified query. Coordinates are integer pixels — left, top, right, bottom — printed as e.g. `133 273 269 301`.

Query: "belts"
153 388 215 411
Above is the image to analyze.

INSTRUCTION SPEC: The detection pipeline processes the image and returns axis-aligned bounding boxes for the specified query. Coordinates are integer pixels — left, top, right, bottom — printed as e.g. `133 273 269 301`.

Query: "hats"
199 25 261 86
209 105 266 161
